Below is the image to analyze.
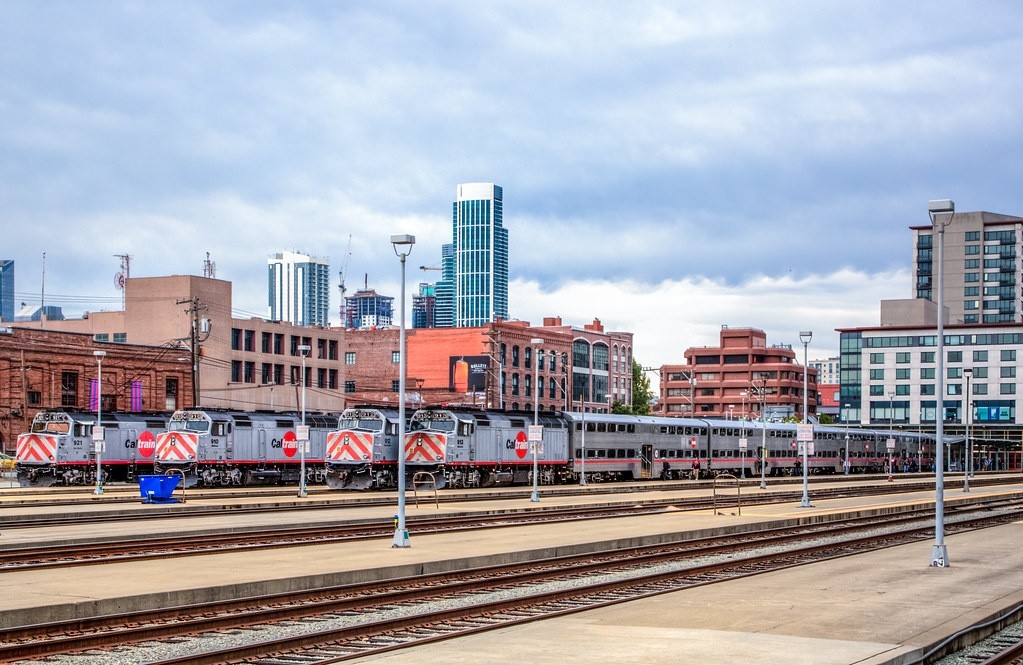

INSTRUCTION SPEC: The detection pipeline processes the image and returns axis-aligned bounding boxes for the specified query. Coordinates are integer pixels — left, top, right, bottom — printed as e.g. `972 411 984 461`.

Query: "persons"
661 457 672 480
793 458 802 476
840 455 1003 474
691 457 700 480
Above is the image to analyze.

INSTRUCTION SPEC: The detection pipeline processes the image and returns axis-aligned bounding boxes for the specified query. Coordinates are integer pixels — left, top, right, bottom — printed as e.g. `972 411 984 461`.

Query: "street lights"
729 404 735 421
963 368 975 492
415 378 425 411
296 344 312 498
816 412 822 424
92 350 108 493
798 330 814 508
605 395 613 414
759 372 771 489
930 199 956 570
845 403 851 476
887 391 896 482
389 233 415 548
529 337 546 502
739 392 748 480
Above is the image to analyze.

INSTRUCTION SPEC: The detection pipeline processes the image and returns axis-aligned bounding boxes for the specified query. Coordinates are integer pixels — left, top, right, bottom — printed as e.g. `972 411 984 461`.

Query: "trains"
398 403 972 491
321 402 418 491
12 407 177 488
153 406 344 490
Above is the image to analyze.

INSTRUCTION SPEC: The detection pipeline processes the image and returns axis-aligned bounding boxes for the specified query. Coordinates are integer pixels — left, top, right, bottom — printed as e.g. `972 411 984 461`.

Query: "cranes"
337 234 356 328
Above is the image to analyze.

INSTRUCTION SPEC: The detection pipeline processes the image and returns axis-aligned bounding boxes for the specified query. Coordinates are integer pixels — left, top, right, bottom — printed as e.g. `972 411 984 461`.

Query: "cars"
0 449 18 469
